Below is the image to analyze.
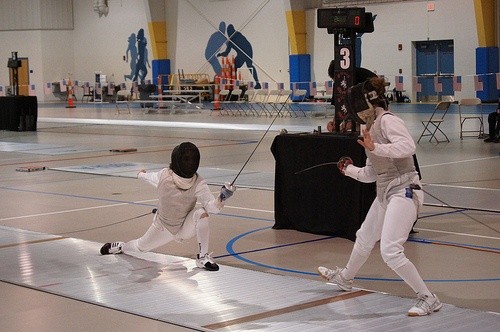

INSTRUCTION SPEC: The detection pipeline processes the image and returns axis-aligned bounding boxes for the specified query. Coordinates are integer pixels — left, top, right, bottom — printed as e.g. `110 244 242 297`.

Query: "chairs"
114 90 132 116
209 88 307 119
81 87 93 103
416 101 452 145
457 98 485 140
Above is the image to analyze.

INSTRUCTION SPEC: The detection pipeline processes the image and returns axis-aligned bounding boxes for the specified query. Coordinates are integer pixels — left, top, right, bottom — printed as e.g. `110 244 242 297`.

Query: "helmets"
344 68 389 124
169 142 200 178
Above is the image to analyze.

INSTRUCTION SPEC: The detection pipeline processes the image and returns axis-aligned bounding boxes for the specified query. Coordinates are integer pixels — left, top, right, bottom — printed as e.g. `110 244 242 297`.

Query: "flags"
248 81 253 89
220 78 226 90
108 83 113 94
29 84 37 96
60 80 66 92
121 83 126 90
85 82 89 93
394 73 500 92
262 82 268 89
277 83 284 92
96 83 102 94
293 81 332 96
234 80 239 90
132 81 138 93
73 80 78 93
44 82 52 95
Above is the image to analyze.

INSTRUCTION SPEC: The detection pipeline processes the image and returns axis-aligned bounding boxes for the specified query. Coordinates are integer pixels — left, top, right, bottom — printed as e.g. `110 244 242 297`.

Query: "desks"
134 100 181 113
149 94 199 108
163 89 209 105
0 94 38 132
270 133 423 243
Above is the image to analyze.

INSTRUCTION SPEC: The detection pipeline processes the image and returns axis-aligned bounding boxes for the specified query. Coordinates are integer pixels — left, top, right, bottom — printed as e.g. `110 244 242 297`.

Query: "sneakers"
196 251 219 271
408 293 442 316
317 266 354 291
100 242 124 254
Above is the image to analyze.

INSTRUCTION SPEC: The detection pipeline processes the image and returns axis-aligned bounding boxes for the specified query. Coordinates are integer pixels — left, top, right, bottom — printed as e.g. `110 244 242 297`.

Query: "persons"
483 96 500 143
317 76 441 315
100 142 225 271
327 60 378 131
